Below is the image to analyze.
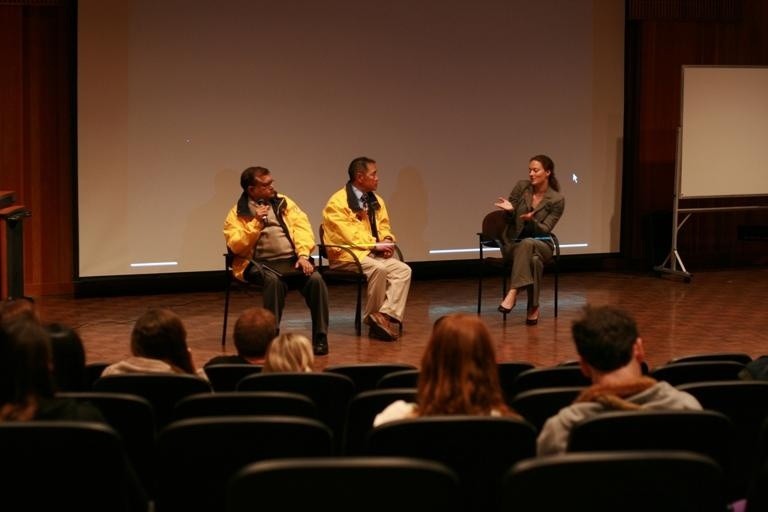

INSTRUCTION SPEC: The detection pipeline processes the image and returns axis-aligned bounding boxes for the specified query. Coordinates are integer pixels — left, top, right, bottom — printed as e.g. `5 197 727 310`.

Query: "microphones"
257 197 268 225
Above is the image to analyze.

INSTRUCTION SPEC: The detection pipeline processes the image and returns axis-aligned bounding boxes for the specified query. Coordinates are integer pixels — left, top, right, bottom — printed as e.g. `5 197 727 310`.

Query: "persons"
221 166 330 356
495 154 565 323
725 417 768 511
262 332 315 373
371 312 527 428
204 308 276 363
100 307 200 377
0 295 107 424
320 157 412 340
737 355 768 381
533 307 704 455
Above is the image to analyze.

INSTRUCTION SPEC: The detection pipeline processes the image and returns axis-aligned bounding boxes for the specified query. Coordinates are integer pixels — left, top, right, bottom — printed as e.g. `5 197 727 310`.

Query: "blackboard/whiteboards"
673 65 768 200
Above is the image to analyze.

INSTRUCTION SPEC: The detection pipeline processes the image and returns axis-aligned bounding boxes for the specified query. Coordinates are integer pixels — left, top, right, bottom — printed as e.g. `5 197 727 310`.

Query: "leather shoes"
498 303 515 313
367 313 399 341
312 334 329 355
527 307 540 324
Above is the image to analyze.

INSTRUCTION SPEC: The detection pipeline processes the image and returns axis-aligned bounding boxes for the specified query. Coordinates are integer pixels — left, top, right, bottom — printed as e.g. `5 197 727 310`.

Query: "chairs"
221 245 315 346
477 211 560 321
317 224 404 337
0 353 768 512
